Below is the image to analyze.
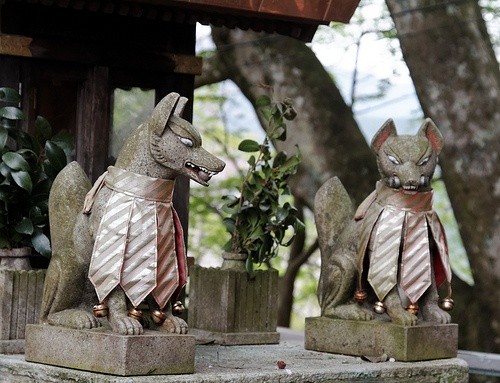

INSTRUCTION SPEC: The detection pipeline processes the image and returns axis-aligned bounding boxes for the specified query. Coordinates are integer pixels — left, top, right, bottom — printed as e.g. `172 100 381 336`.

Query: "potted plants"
0 87 74 270
216 93 306 274
